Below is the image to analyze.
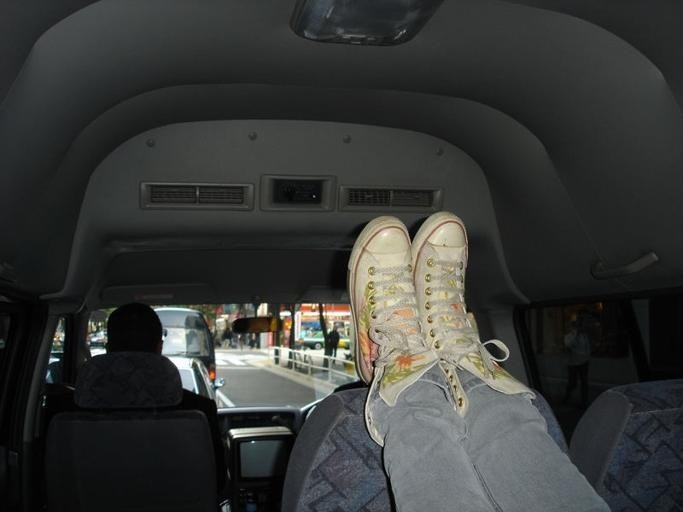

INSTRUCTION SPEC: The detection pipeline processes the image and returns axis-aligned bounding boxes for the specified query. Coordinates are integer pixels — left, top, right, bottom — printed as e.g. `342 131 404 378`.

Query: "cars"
40 309 224 406
300 329 350 349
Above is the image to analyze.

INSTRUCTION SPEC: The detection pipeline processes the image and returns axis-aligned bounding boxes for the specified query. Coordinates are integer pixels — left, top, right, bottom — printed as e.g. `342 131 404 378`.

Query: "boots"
410 211 534 420
346 214 457 449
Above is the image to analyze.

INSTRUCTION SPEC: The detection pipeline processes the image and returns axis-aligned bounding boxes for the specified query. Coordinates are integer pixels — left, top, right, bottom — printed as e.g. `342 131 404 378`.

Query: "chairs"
279 386 571 511
41 351 226 512
564 380 682 511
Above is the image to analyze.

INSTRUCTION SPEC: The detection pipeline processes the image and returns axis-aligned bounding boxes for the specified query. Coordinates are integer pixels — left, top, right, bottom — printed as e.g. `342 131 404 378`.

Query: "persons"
347 206 612 512
327 325 340 363
40 301 228 500
